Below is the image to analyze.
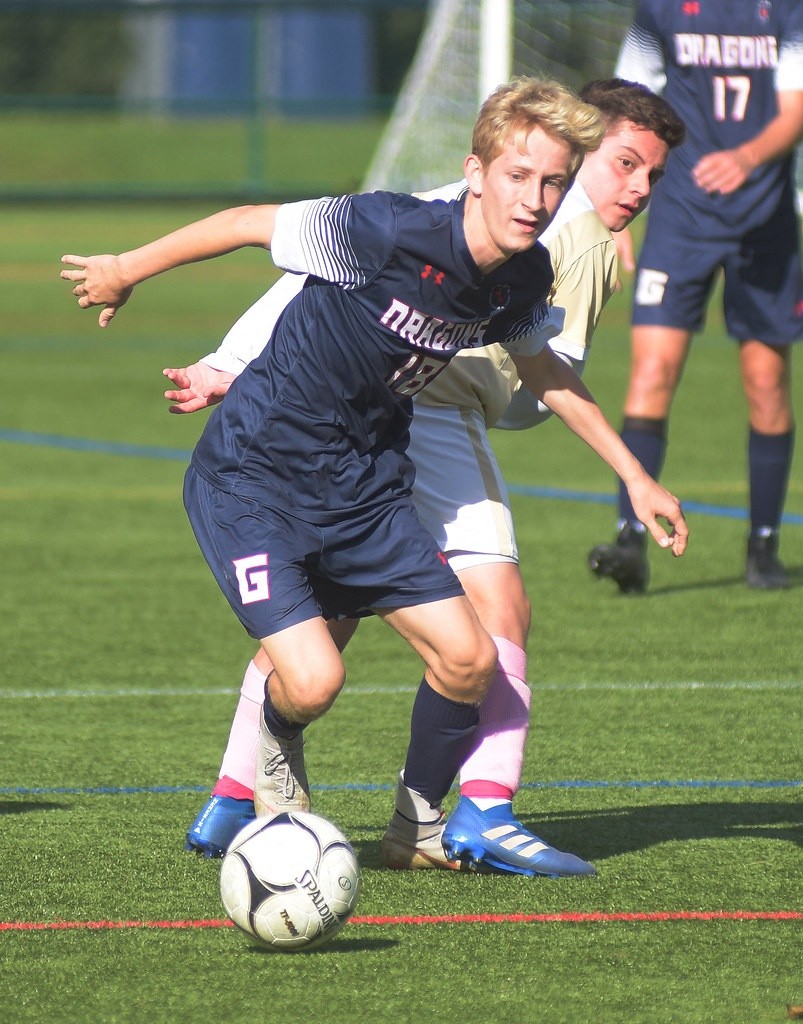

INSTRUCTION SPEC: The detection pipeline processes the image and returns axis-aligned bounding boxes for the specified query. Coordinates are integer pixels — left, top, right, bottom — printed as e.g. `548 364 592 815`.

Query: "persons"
590 0 803 594
59 78 690 871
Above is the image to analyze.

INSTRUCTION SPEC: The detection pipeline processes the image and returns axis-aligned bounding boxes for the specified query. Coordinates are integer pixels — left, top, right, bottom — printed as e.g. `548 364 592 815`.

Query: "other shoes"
588 544 649 593
747 548 791 589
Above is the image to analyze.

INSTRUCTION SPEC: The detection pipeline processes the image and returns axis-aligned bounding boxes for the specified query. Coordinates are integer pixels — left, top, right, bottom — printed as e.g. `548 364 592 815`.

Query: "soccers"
218 810 363 953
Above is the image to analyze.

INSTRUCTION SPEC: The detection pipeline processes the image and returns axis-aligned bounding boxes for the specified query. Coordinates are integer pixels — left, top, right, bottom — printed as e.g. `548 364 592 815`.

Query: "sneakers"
441 796 595 877
184 796 255 856
254 701 311 818
381 826 498 873
